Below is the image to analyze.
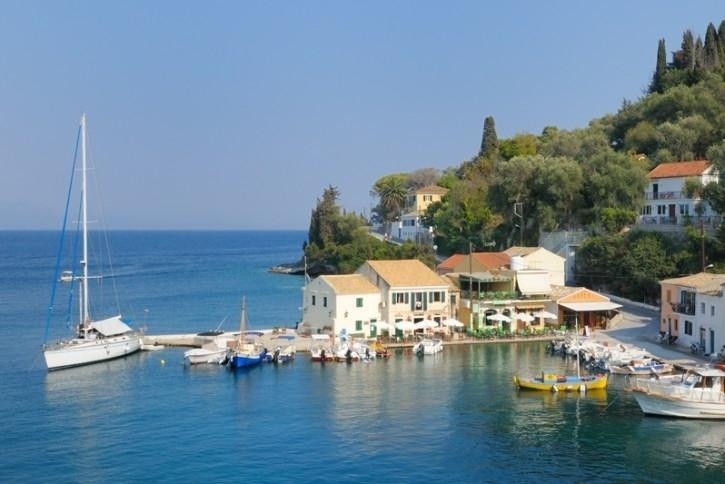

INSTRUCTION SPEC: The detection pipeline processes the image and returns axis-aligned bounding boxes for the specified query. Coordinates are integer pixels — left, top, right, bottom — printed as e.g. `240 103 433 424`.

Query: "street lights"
413 216 437 257
704 264 714 273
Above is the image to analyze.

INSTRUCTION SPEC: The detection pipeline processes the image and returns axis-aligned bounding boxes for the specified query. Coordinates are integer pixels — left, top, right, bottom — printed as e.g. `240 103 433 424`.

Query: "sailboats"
265 335 296 365
512 315 609 392
42 112 142 372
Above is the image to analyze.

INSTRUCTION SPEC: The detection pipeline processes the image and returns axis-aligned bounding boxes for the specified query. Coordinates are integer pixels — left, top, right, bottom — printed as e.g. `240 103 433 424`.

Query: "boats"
184 336 227 367
631 364 725 421
57 270 77 282
543 328 694 373
229 296 267 368
307 333 392 360
412 338 443 357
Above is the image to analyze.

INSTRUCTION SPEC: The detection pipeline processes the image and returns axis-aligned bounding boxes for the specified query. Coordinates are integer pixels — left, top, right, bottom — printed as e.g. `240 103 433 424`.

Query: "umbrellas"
368 310 558 341
